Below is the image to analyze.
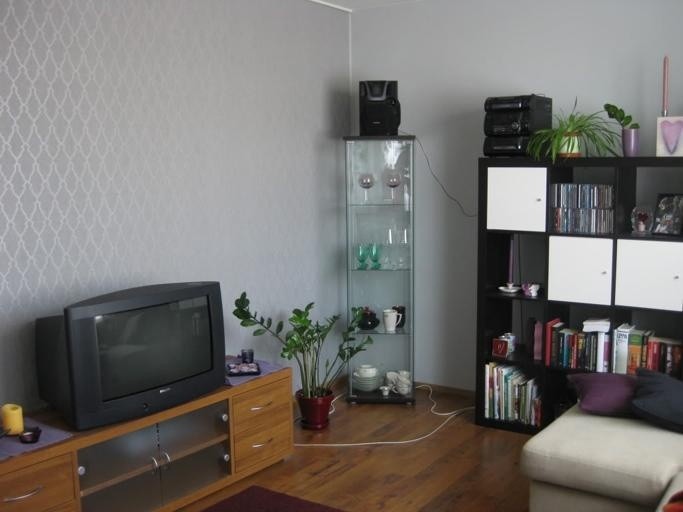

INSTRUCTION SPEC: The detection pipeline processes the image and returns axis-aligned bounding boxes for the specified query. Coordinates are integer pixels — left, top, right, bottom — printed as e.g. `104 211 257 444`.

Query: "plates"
498 286 521 293
352 372 384 392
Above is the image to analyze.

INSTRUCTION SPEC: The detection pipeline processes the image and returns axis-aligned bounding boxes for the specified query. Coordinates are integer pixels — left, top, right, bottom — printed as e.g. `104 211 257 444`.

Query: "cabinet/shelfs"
343 140 411 402
479 161 682 431
234 377 294 470
1 451 76 509
76 399 228 512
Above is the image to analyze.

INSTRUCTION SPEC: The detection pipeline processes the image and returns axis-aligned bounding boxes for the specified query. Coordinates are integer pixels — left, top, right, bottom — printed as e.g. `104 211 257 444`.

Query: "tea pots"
355 365 378 377
357 306 380 330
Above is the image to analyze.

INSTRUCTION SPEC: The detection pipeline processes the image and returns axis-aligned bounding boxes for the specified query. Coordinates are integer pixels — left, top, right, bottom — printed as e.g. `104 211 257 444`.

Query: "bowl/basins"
19 428 42 444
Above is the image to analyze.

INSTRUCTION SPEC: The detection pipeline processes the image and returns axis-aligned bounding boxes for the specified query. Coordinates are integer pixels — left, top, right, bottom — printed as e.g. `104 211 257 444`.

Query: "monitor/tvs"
37 282 226 433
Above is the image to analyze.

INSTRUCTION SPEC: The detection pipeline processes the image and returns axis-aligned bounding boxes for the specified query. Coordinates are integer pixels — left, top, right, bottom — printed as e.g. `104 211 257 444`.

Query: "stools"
521 401 683 512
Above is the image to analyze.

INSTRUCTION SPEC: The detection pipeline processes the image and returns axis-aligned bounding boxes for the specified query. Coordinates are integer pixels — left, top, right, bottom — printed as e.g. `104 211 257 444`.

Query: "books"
484 361 541 428
532 318 683 377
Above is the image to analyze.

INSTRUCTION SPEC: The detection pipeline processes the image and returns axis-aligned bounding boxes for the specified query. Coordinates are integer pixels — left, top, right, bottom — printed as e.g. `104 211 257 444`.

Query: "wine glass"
357 173 375 204
383 168 403 202
353 226 409 270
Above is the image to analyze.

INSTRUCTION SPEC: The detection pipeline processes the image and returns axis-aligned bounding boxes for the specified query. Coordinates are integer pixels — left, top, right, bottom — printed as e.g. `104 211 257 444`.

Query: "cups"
392 306 406 328
382 309 402 331
522 283 540 297
507 282 513 289
1 402 24 437
242 349 254 364
622 129 640 157
380 369 411 395
227 361 258 374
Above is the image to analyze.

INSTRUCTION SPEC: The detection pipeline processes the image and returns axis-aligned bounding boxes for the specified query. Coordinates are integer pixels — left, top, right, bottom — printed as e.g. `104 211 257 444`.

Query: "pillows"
566 372 634 415
632 367 683 431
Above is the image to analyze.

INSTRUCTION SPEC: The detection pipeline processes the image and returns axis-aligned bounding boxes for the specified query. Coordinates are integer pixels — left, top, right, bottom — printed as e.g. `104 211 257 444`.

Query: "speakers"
357 81 400 134
483 95 553 157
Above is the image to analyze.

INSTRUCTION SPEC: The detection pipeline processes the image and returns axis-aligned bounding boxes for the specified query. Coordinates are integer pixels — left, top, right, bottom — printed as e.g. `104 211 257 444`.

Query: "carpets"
202 483 340 511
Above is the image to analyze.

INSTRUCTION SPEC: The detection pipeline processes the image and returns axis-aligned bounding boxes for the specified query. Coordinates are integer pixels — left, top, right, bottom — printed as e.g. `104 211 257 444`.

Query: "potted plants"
530 108 614 156
604 103 640 156
235 291 369 433
